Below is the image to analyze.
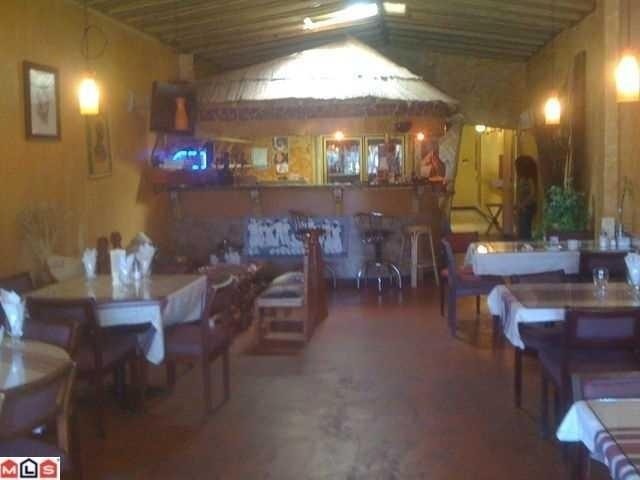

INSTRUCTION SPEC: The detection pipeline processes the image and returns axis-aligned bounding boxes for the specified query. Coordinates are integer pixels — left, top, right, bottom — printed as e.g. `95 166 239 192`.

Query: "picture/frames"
23 60 63 140
86 112 113 180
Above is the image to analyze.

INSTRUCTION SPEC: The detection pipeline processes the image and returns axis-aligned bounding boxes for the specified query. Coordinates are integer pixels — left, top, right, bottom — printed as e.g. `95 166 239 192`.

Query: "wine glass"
593 266 609 300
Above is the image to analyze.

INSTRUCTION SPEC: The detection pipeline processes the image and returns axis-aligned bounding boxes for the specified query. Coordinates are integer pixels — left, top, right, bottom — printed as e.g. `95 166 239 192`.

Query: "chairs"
286 209 337 290
353 208 403 294
440 236 506 338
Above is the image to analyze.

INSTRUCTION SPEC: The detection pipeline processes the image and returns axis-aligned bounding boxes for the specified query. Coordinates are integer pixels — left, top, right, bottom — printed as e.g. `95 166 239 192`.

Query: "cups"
567 239 578 250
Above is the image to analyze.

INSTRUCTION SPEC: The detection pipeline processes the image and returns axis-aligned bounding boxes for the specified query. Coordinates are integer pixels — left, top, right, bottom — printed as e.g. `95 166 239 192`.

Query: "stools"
399 223 440 289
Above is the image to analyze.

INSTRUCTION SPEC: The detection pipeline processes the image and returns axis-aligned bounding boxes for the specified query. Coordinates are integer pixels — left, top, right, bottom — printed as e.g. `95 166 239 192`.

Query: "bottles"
599 231 608 250
610 239 616 249
132 260 141 280
216 150 249 176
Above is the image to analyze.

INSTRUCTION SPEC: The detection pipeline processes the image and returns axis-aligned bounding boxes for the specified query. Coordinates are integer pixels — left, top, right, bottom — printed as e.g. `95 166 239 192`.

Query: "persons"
514 156 537 243
273 136 289 175
